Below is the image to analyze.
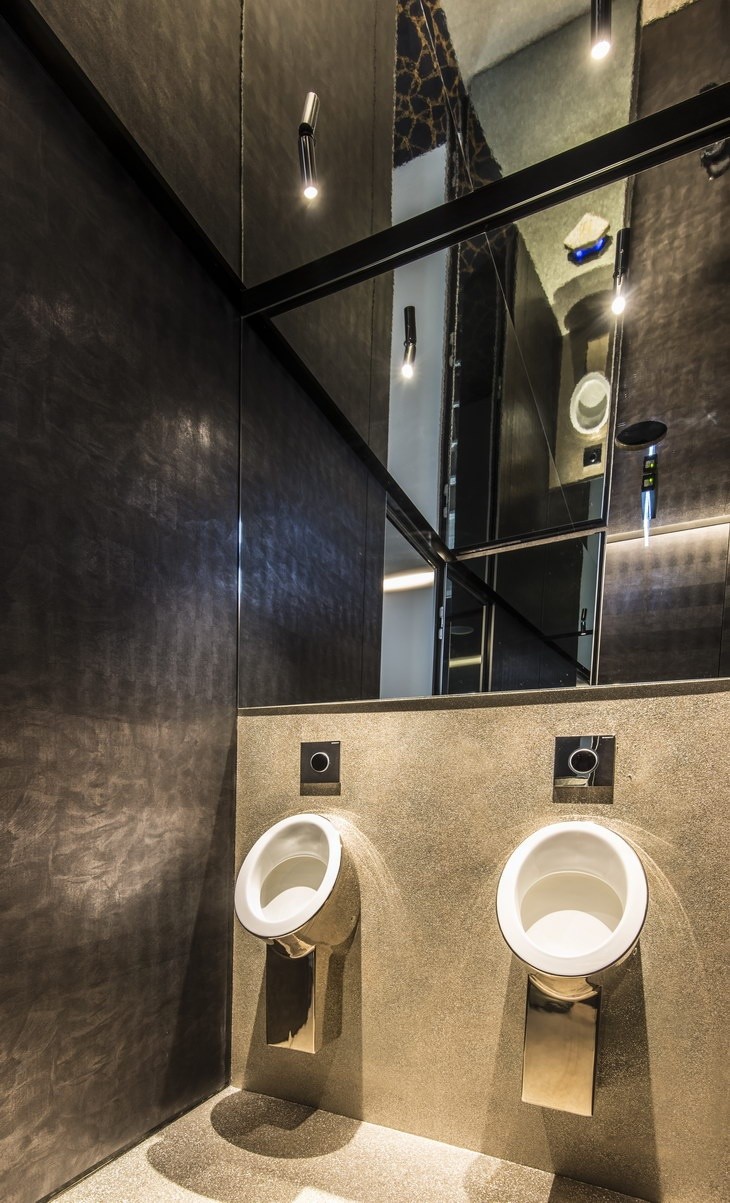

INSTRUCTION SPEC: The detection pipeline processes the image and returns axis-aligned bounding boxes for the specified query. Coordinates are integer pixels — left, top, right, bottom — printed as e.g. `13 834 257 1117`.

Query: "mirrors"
235 130 730 714
237 0 728 288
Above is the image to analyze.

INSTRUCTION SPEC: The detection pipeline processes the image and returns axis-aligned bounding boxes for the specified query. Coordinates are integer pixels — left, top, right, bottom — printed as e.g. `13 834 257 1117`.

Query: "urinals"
233 812 360 947
567 372 611 436
495 822 652 984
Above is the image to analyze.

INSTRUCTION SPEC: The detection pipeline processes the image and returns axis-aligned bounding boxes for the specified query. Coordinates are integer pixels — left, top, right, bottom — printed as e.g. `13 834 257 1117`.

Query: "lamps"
296 91 319 135
590 0 611 62
297 135 321 201
403 306 416 345
402 342 417 378
